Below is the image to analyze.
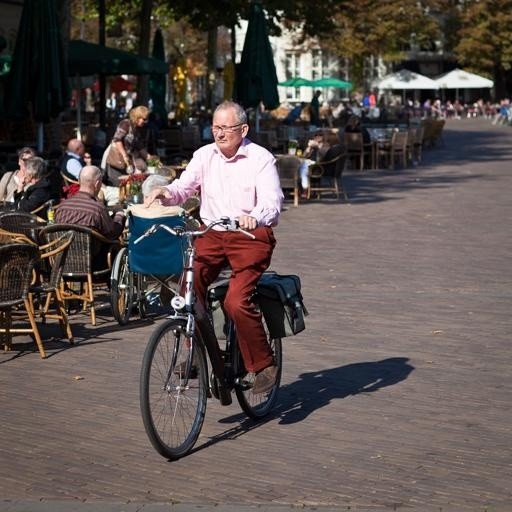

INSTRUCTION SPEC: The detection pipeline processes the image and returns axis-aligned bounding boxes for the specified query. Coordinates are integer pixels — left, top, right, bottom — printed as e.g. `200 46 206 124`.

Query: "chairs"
59 156 201 324
262 117 447 209
2 243 47 360
30 199 56 224
39 222 126 325
1 211 64 317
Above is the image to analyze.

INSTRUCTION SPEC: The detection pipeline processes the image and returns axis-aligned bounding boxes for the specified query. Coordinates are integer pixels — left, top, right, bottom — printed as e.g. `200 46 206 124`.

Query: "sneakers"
252 361 279 393
174 360 200 374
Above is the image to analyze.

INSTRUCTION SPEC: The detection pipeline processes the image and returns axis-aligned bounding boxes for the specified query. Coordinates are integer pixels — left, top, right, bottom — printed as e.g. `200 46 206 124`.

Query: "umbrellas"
6 1 78 158
0 35 170 128
230 5 281 141
148 26 169 127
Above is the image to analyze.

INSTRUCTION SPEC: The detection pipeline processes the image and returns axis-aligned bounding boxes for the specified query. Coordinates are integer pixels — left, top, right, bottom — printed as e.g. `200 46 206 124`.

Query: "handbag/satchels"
258 273 306 338
107 147 125 169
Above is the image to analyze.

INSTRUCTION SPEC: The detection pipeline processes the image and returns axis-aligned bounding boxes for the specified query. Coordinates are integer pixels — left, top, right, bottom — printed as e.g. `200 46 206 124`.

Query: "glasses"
211 124 243 131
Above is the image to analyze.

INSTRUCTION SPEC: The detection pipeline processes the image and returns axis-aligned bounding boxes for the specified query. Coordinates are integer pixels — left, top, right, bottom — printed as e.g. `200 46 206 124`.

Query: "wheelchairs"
111 210 211 326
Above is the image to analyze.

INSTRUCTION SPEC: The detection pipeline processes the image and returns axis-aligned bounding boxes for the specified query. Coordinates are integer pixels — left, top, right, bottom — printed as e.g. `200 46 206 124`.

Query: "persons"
60 138 93 187
325 87 511 128
342 113 372 173
100 105 163 186
302 129 325 163
12 155 53 214
307 90 325 128
0 146 38 204
99 142 114 176
299 132 344 199
143 100 286 395
52 164 126 273
282 100 307 125
123 174 190 307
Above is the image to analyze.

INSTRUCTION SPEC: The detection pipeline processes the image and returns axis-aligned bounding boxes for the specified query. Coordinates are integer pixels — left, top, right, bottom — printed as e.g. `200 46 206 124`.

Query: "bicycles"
134 218 305 458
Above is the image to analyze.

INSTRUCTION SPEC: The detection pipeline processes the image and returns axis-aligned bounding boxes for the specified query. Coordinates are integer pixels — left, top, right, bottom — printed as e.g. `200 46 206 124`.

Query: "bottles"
297 149 302 157
47 199 55 224
